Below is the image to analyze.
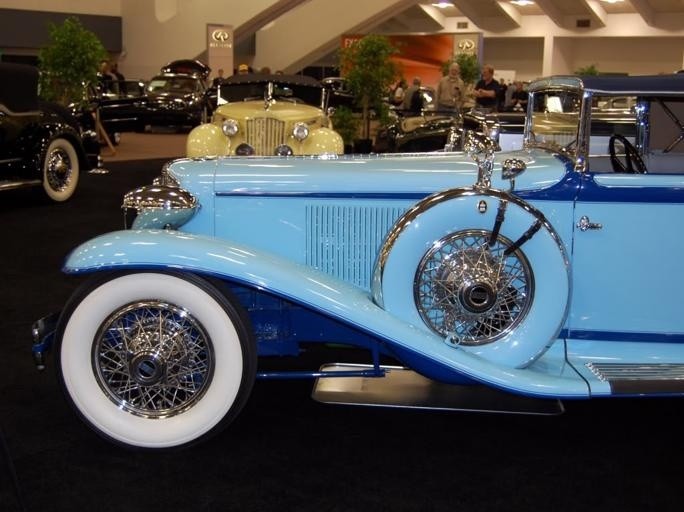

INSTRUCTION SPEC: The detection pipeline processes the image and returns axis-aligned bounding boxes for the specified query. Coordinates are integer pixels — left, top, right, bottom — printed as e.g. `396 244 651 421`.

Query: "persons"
212 67 227 90
238 65 249 78
97 60 118 95
391 78 409 107
433 62 464 119
108 61 128 95
464 63 500 115
400 76 421 117
274 70 284 76
498 78 529 114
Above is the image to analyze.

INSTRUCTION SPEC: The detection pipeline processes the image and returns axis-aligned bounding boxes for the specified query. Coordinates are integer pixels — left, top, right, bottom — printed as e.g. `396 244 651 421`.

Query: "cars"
0 55 211 202
